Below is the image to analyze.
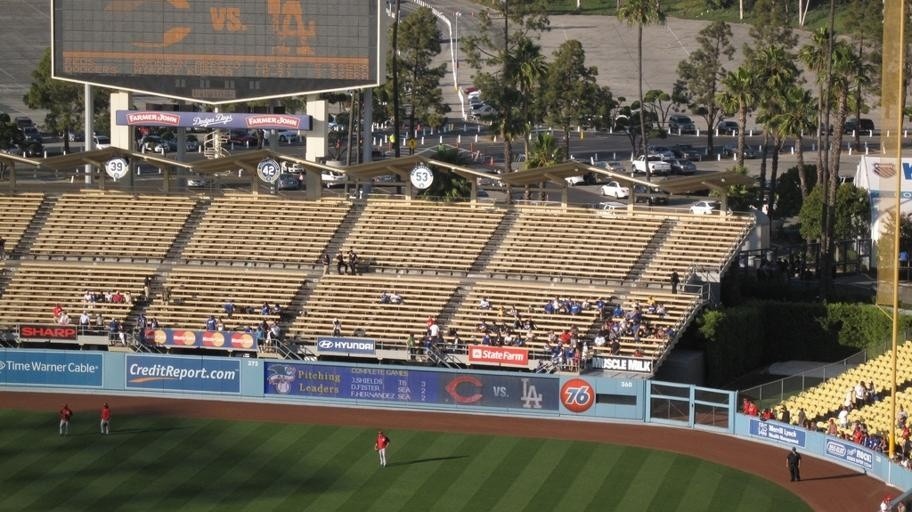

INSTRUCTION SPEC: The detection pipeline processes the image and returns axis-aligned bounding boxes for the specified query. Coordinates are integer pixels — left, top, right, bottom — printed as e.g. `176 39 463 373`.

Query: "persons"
742 380 912 512
375 292 403 305
321 249 359 276
100 402 112 435
406 295 680 374
58 403 73 436
207 302 281 353
375 430 390 468
51 273 171 352
671 271 679 294
332 319 365 337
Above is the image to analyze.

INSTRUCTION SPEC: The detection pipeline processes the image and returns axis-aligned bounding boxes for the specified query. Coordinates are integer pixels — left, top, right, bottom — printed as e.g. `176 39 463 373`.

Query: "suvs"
468 91 505 121
614 110 653 134
668 115 696 134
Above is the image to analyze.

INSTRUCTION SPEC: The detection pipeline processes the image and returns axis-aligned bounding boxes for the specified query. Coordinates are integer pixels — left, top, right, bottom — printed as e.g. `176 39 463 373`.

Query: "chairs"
761 338 911 466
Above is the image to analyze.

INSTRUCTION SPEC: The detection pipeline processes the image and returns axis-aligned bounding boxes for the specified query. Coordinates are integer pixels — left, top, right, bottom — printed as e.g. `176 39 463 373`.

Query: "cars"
134 160 164 176
278 161 348 191
564 142 779 217
56 128 214 153
718 121 739 135
0 111 51 158
327 113 420 132
233 128 297 146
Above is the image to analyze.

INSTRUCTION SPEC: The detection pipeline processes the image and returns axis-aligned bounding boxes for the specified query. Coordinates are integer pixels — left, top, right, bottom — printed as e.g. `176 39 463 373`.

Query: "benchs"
1 187 757 365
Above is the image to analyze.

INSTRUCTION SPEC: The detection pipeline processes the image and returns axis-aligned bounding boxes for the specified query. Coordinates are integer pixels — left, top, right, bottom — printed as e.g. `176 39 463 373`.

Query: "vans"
844 118 874 136
